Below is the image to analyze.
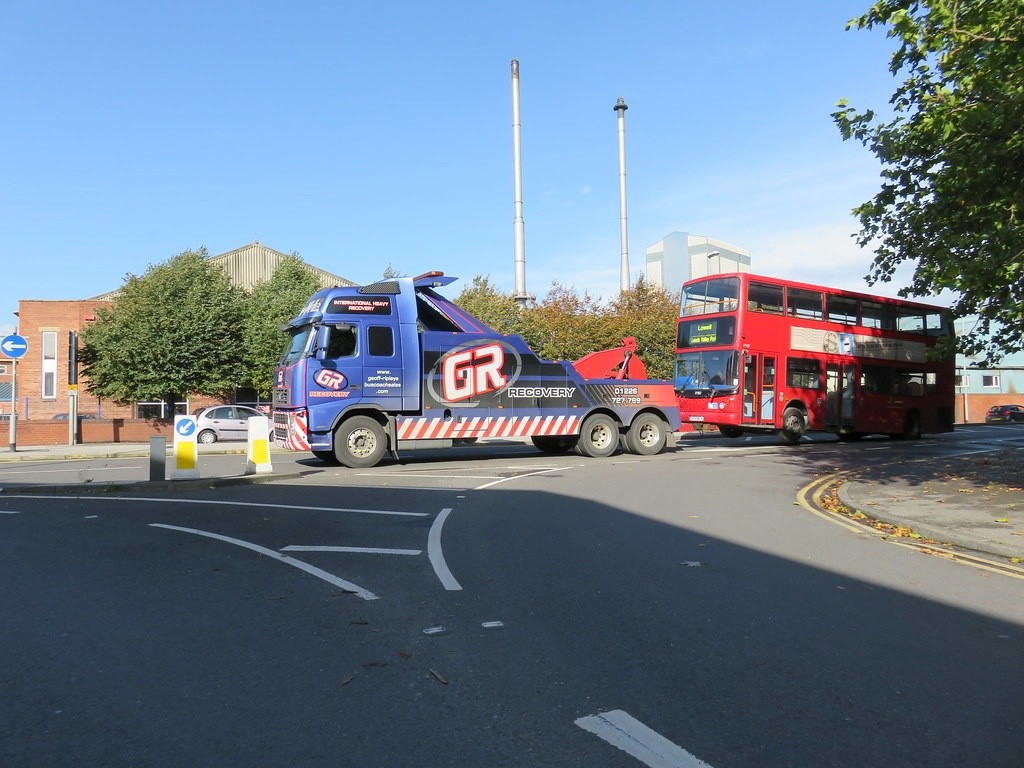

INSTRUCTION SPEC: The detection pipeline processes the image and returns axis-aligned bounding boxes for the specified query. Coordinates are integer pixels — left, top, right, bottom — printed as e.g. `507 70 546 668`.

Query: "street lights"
708 250 721 275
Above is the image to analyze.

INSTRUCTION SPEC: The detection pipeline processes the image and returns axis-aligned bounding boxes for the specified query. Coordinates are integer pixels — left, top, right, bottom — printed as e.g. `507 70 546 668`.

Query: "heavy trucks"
269 269 683 471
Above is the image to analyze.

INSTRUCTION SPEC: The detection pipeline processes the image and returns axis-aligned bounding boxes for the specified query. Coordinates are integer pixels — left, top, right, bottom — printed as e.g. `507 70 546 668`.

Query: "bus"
676 272 957 444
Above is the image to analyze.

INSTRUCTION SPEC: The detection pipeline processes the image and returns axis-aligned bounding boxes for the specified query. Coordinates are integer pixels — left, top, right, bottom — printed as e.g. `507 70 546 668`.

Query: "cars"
187 404 276 445
984 404 1024 426
